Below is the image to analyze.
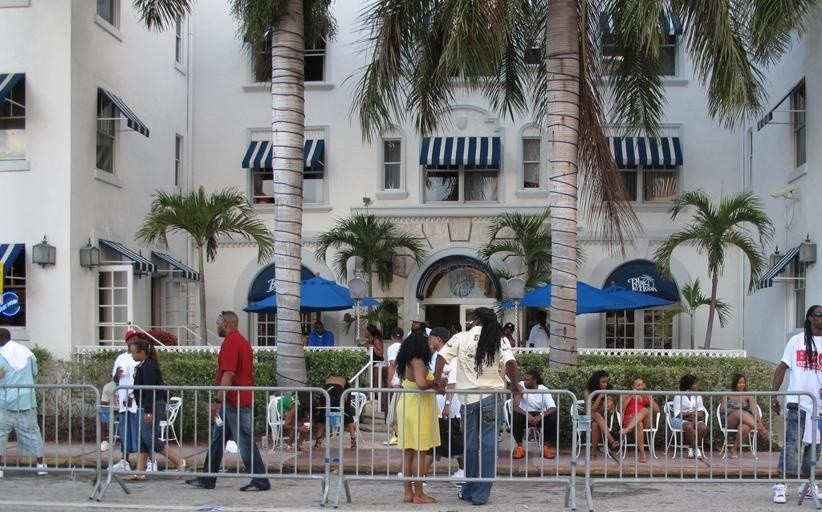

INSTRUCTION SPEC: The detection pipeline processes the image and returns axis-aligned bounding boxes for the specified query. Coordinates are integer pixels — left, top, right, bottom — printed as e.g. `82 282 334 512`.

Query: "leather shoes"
185 479 216 489
240 484 272 491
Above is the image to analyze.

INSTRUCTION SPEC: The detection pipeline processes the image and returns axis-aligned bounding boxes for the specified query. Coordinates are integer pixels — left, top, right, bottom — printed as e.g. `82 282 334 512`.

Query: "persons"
314 376 357 450
301 326 307 347
277 391 317 453
308 322 334 346
673 375 708 459
359 307 558 506
618 374 660 463
0 329 49 478
185 312 270 491
771 305 822 503
99 330 188 481
720 374 771 460
583 371 620 461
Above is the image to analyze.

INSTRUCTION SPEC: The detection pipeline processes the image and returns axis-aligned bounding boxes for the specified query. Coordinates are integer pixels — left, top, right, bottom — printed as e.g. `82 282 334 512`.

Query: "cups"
261 435 269 450
304 422 310 431
119 367 126 375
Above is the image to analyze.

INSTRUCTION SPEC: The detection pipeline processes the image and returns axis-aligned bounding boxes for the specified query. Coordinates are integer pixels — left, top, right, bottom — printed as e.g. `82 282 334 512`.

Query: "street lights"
348 270 372 347
506 274 529 347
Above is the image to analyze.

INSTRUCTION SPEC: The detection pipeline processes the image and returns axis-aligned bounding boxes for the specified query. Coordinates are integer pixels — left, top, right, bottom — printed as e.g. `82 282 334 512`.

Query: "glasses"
218 310 227 321
810 311 822 317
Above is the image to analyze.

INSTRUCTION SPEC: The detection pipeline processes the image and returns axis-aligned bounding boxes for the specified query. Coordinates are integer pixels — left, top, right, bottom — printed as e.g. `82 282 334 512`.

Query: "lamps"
79 238 100 270
769 246 786 275
798 232 817 267
347 278 367 298
32 234 56 268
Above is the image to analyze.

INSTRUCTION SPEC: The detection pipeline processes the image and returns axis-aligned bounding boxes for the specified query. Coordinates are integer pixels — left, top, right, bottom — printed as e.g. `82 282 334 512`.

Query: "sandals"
313 435 324 450
402 493 439 504
350 436 358 450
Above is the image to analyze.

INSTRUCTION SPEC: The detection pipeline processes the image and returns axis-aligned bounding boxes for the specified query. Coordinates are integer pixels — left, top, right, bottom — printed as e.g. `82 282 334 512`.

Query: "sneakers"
798 482 822 500
456 490 487 506
100 440 109 451
450 468 466 478
771 482 788 503
382 432 399 445
687 446 703 460
512 446 525 459
106 458 131 471
619 428 628 439
35 462 49 476
286 444 309 453
398 470 428 487
146 457 158 472
540 442 556 459
0 470 5 478
120 474 146 481
172 458 187 480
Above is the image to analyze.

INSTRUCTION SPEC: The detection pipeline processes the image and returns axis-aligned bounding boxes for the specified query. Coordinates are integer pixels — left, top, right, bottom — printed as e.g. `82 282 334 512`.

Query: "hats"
124 330 141 341
430 326 449 338
409 319 431 324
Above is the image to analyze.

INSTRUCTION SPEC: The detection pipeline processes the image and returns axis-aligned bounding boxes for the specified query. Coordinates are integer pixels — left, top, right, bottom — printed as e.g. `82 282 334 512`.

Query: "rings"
773 404 775 407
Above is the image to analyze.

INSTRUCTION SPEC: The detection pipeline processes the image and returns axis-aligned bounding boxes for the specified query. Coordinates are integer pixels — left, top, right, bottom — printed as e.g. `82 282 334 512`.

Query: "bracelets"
771 398 776 402
214 398 223 402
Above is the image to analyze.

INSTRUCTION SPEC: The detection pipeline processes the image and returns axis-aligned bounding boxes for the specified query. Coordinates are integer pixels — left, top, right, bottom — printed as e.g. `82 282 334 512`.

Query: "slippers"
639 455 648 463
731 453 738 459
759 430 770 441
608 439 620 451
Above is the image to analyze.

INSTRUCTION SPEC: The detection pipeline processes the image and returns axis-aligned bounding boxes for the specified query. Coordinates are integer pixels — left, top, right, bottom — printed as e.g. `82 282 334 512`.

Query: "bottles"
208 405 224 427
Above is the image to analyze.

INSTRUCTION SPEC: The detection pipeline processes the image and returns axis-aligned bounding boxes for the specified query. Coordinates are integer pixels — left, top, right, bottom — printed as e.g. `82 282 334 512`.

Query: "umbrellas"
602 281 674 347
242 272 380 323
501 281 637 315
